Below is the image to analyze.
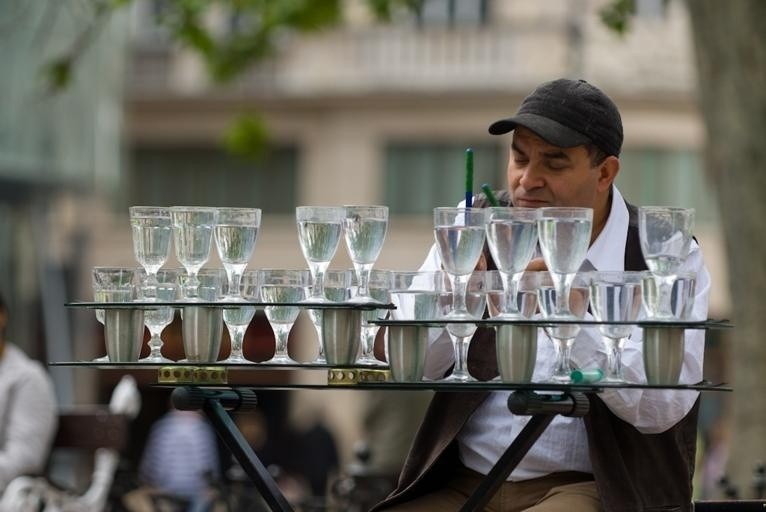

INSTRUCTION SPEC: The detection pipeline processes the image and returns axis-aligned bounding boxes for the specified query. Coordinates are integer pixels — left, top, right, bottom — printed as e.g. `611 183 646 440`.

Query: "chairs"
0 411 128 511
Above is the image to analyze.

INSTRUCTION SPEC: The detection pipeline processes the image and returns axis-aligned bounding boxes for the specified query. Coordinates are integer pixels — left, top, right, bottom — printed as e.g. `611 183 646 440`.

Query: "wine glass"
340 203 389 305
127 201 170 300
171 206 209 304
483 205 536 323
92 273 704 387
215 206 262 306
540 208 595 321
293 203 346 305
431 203 486 323
637 203 690 321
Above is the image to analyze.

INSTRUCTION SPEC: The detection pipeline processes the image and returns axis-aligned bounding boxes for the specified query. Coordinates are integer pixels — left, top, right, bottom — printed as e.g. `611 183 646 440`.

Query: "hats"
486 76 626 159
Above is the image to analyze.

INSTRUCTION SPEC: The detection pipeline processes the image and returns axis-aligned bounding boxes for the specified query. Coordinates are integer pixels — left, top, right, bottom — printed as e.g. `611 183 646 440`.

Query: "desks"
141 384 605 512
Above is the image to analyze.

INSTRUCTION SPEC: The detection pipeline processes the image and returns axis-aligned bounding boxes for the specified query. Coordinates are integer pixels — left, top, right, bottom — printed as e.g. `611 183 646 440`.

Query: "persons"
1 294 60 500
139 401 221 512
369 77 711 512
